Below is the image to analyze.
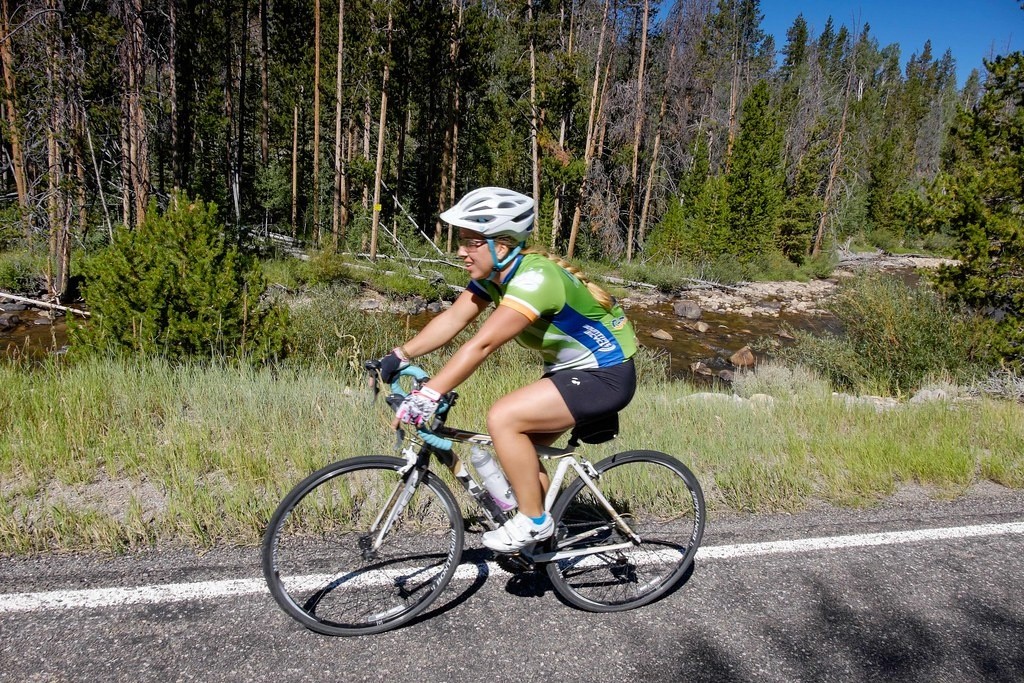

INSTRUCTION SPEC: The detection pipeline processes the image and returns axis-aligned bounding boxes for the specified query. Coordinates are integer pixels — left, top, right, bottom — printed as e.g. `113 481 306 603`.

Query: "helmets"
439 187 535 242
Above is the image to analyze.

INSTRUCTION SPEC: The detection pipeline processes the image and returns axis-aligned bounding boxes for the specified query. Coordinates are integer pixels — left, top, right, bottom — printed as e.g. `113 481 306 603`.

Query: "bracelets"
399 343 410 359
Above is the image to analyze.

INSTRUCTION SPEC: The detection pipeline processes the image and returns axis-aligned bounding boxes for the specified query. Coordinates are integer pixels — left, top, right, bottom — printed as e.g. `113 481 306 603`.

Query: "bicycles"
259 358 706 637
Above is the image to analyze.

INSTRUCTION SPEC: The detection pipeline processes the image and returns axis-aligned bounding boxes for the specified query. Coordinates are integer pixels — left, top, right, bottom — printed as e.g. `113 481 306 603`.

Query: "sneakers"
482 511 555 552
554 521 568 541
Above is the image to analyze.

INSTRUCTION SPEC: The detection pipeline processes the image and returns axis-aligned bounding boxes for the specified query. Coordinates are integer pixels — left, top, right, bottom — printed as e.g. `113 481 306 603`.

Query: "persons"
364 187 642 558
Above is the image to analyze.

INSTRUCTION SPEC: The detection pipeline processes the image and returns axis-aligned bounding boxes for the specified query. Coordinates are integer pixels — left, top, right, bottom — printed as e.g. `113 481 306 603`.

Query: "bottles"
471 446 519 512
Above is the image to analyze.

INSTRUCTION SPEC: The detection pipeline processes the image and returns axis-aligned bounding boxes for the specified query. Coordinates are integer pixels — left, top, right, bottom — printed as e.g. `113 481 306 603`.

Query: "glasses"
456 237 496 251
